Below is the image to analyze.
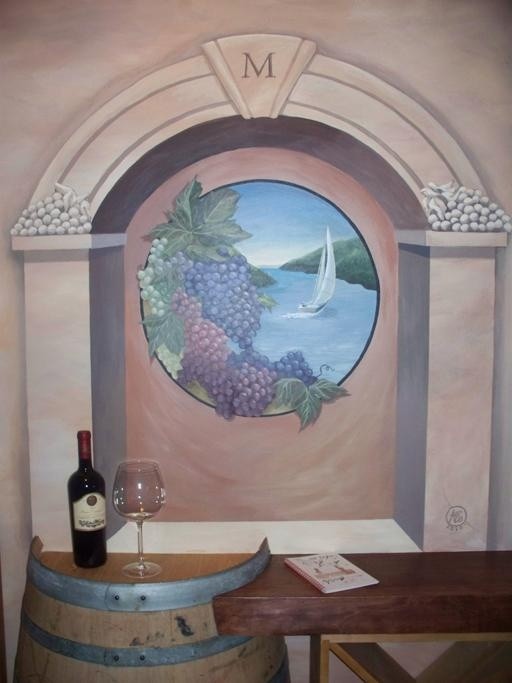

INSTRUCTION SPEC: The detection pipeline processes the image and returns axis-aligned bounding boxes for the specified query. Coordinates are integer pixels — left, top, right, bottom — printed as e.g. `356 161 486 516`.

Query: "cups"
112 461 166 579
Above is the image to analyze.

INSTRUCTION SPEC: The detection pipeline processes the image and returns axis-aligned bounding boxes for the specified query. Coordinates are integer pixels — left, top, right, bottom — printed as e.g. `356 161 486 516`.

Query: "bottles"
67 430 107 567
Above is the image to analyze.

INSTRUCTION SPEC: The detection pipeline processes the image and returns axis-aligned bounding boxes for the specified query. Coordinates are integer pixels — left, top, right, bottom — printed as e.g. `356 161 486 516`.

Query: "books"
283 551 379 594
